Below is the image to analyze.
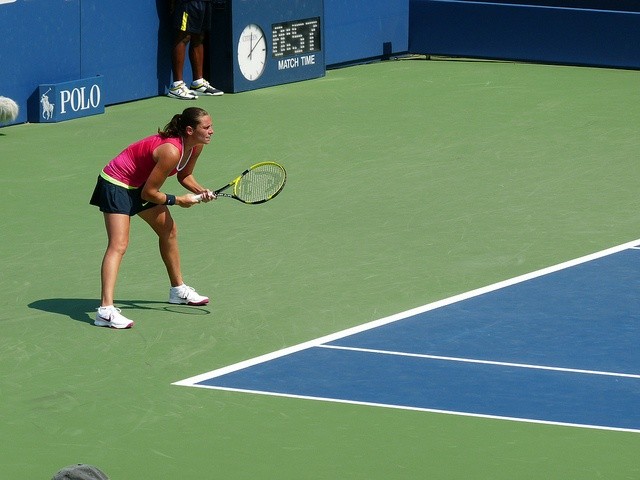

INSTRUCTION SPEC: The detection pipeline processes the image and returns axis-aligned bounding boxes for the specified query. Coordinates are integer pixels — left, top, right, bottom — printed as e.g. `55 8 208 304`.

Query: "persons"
89 107 217 330
167 1 224 101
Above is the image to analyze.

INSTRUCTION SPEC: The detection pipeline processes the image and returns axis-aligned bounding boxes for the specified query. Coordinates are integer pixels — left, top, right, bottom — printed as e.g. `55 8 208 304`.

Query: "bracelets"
162 193 175 206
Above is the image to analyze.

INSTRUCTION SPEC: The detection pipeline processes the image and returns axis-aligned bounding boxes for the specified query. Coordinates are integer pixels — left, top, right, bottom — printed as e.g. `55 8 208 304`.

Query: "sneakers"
94 306 135 328
168 82 198 100
190 80 224 96
169 283 209 306
